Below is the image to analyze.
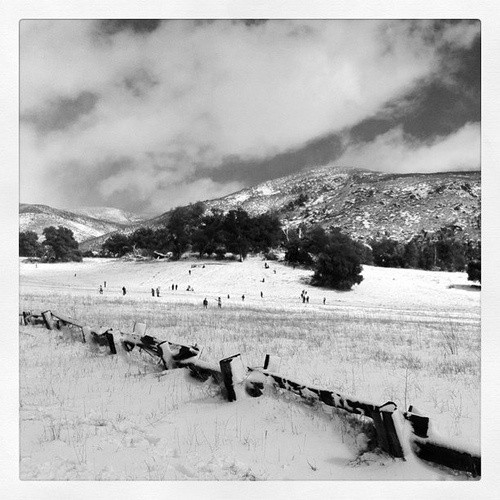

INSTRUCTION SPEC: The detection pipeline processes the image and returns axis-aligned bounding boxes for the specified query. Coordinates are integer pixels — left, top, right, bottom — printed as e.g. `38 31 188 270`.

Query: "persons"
74 260 329 310
